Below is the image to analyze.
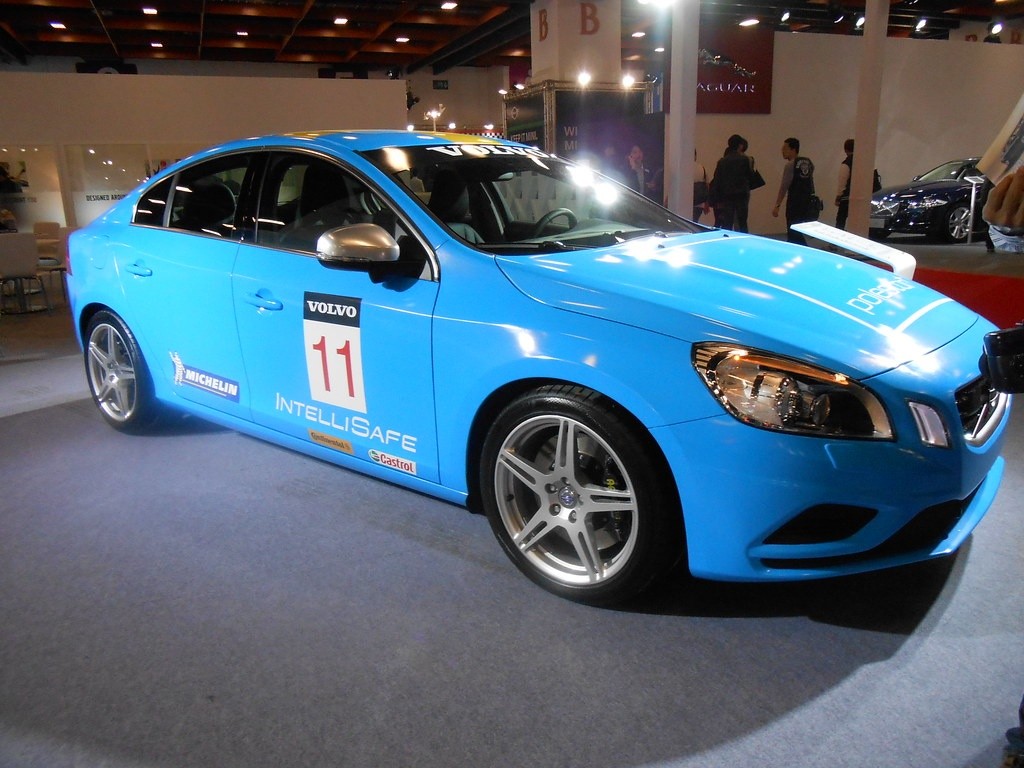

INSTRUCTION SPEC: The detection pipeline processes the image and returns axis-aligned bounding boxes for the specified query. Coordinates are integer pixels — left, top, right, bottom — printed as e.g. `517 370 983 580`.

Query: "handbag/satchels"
748 157 765 191
808 194 820 222
873 169 882 194
693 166 708 207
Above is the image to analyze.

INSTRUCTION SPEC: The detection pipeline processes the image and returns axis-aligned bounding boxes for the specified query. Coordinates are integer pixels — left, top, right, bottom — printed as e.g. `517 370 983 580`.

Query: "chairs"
418 167 485 244
0 222 74 315
300 165 409 250
179 180 238 237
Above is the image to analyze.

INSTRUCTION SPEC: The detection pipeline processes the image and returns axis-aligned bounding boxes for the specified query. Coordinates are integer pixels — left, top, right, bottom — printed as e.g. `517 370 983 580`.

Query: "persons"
772 137 816 247
693 148 710 223
600 143 625 188
709 134 755 235
623 144 656 198
823 138 854 251
981 164 1024 253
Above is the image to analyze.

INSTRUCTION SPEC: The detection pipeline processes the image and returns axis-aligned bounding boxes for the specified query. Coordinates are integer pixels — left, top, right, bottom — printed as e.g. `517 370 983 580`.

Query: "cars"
64 128 1015 606
869 156 994 245
